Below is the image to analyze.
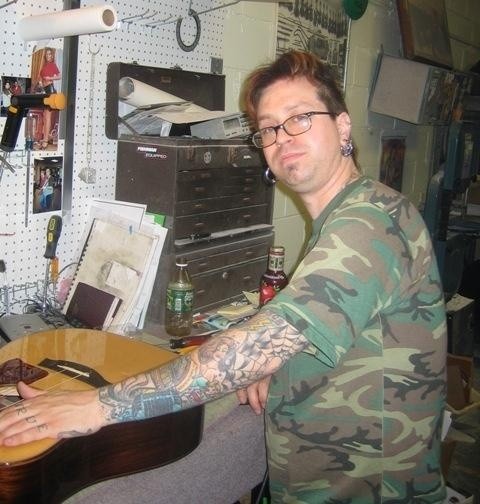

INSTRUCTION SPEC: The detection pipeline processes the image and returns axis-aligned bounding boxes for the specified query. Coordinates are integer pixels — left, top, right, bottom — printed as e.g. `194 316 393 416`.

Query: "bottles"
259 246 288 308
164 256 195 336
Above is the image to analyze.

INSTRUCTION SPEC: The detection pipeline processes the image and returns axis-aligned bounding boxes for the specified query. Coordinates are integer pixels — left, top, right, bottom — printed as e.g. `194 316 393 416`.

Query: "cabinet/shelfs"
105 64 276 325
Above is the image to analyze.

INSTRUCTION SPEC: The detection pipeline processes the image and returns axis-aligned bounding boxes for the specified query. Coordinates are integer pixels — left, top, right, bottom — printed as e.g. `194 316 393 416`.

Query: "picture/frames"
398 0 453 70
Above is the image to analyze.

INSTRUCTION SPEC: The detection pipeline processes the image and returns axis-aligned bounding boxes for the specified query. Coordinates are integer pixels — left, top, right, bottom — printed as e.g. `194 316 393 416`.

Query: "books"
59 195 168 337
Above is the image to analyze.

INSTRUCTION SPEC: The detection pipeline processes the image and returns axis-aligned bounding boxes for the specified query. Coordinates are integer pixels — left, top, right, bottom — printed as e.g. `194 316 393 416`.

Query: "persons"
43 169 55 211
0 82 13 116
39 170 46 211
11 80 21 95
41 49 61 150
0 52 448 504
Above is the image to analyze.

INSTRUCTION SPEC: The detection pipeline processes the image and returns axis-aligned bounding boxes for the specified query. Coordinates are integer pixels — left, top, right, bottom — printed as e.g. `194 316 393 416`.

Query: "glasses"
250 111 336 149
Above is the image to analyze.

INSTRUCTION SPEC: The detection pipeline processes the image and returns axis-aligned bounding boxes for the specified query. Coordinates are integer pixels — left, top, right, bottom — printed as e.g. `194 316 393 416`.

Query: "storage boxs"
367 51 466 126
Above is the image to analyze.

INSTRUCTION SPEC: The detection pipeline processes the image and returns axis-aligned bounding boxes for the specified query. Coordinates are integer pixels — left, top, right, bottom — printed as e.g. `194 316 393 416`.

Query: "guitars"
0 327 203 501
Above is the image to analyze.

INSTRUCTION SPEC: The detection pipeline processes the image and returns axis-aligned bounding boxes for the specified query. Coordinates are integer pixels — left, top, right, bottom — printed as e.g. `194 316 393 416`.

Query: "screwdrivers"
42 215 62 313
157 334 213 349
